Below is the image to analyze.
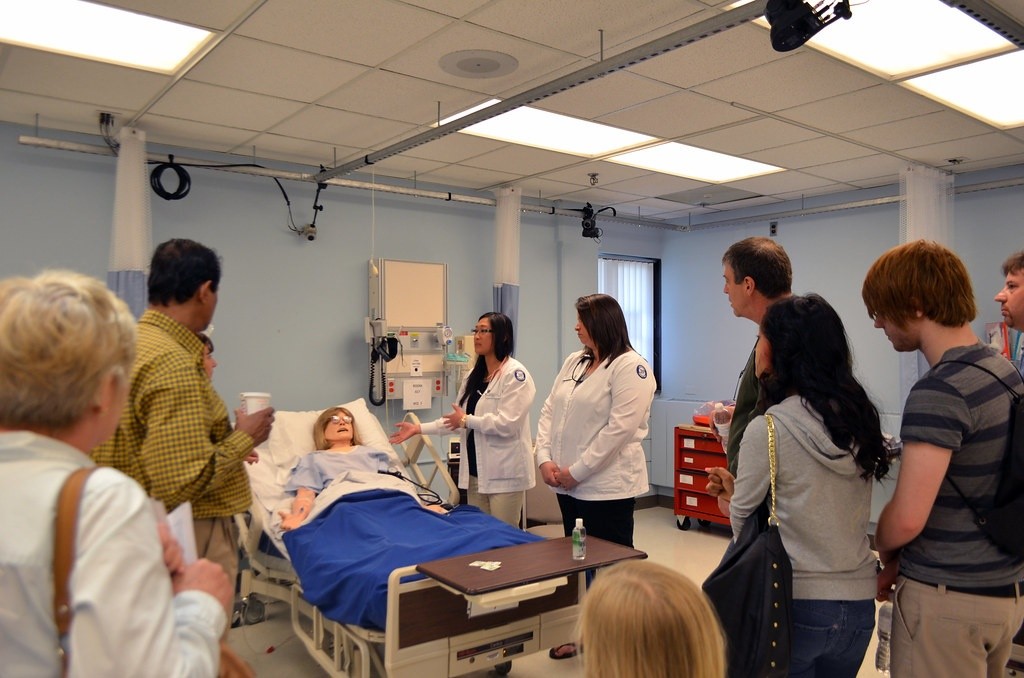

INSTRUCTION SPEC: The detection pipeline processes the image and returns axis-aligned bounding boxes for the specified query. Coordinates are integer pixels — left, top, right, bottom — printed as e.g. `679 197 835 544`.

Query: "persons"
722 238 793 478
0 271 234 678
707 293 891 678
862 240 1024 678
278 407 443 530
533 294 657 660
89 238 274 640
389 312 536 529
995 250 1024 332
577 559 726 678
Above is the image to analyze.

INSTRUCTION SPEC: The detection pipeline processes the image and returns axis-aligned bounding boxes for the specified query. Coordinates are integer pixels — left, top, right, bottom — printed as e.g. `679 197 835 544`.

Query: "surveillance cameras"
582 220 599 238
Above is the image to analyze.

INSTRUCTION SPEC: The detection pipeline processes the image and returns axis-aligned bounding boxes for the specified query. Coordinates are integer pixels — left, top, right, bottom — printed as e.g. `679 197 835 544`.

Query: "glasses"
329 415 352 424
470 328 492 335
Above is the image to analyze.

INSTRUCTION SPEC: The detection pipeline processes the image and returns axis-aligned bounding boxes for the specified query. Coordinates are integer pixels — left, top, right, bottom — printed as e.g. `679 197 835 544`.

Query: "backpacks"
932 360 1024 558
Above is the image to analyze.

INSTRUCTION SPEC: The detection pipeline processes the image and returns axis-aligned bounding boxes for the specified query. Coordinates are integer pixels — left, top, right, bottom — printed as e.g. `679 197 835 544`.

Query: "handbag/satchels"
702 414 791 678
50 466 256 678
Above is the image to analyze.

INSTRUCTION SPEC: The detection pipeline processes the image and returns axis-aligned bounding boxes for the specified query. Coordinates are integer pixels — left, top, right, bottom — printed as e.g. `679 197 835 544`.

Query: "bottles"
875 590 894 674
713 402 732 454
572 518 586 560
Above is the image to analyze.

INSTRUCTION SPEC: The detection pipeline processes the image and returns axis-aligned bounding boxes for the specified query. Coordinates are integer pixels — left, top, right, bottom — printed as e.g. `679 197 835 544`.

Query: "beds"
235 408 650 678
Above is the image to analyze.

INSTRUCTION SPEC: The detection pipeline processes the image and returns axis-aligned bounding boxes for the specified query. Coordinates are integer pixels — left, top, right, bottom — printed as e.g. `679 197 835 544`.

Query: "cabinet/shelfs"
674 426 738 533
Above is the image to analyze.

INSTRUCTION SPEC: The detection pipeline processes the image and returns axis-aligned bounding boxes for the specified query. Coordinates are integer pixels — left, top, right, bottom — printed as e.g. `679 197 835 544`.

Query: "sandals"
549 642 582 658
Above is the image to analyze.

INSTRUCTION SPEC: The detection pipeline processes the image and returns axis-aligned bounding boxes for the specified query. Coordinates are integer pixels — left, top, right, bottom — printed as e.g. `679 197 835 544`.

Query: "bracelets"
460 414 467 428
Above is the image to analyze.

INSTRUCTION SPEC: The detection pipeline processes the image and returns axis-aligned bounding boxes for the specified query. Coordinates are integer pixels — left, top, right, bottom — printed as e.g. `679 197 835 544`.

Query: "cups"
242 392 271 416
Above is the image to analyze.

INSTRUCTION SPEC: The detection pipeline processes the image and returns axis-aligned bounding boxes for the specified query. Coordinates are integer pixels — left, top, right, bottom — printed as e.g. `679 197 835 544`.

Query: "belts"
898 572 1024 599
